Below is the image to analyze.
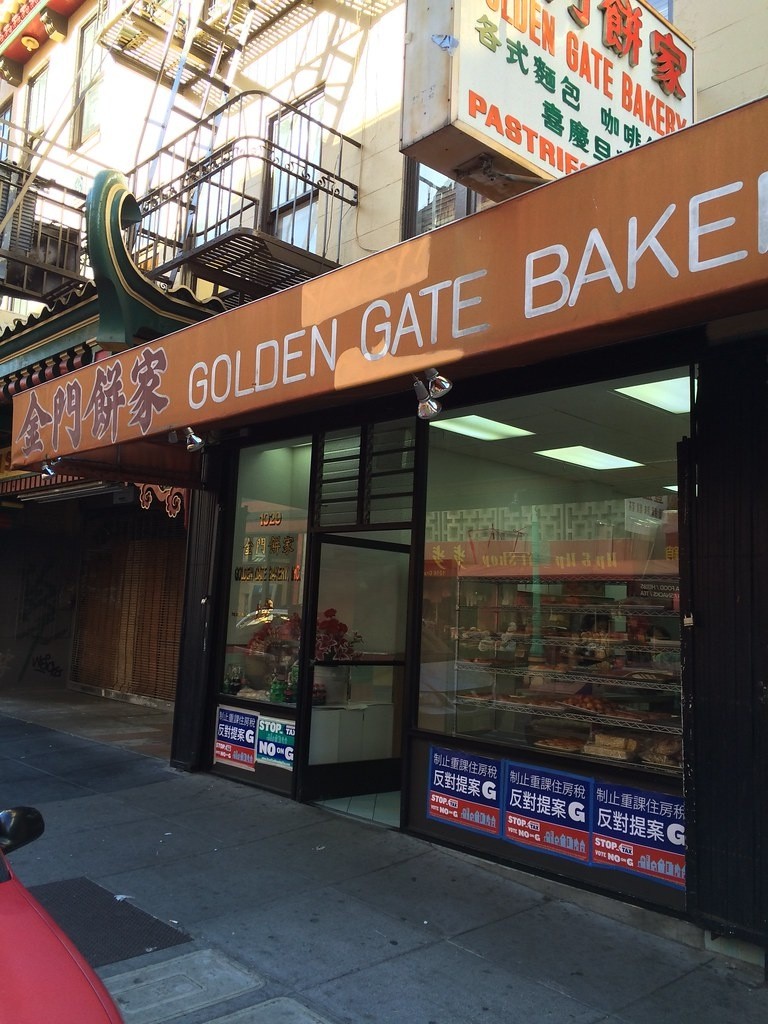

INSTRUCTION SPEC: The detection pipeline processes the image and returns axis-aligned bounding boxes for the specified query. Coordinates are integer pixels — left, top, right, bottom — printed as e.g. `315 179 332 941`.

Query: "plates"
456 603 684 771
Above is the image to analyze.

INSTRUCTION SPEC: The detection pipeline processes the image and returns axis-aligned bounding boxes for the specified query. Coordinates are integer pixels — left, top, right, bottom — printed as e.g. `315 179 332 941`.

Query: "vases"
312 664 349 705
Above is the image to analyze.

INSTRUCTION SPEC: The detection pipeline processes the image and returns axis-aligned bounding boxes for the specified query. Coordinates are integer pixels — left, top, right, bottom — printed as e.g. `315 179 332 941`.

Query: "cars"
0 804 126 1024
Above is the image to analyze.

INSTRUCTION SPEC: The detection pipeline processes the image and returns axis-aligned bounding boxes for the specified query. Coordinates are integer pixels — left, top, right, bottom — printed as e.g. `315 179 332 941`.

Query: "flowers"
247 606 365 661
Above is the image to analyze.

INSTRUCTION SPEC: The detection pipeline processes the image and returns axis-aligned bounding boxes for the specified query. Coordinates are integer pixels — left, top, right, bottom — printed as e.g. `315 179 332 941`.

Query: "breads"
458 657 682 768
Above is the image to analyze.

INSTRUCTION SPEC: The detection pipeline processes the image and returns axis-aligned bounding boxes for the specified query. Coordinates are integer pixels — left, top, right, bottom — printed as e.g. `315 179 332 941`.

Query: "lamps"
409 374 443 420
167 426 205 453
424 367 452 399
40 457 62 479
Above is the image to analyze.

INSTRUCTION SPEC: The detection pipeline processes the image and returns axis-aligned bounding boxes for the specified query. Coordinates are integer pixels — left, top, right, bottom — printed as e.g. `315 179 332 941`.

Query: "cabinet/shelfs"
226 640 406 704
452 562 684 781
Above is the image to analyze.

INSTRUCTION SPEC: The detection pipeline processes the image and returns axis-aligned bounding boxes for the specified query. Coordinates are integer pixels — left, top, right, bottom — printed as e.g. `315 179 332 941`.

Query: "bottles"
270 673 327 706
223 672 248 694
